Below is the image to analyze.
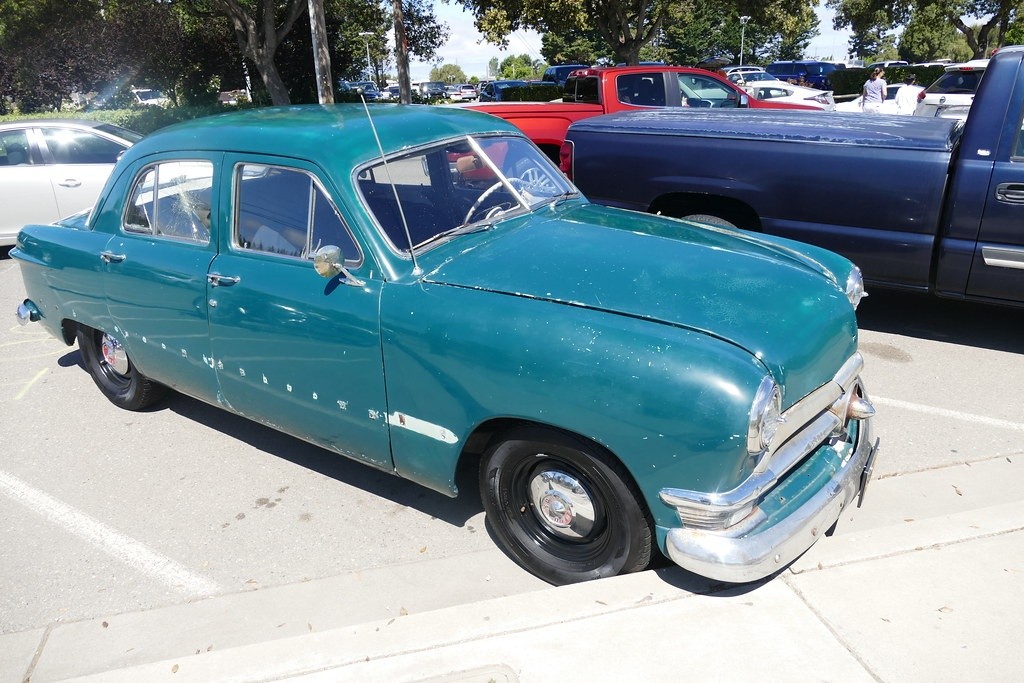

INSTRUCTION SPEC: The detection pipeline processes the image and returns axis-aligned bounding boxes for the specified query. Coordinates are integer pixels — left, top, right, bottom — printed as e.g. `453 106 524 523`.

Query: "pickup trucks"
556 45 1024 321
423 65 827 192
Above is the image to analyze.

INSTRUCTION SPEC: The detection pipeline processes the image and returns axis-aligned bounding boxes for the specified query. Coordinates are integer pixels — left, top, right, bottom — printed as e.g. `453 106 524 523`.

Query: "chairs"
634 79 655 106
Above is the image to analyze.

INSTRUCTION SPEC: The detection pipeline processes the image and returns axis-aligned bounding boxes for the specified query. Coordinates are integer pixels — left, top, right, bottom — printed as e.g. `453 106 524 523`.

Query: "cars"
479 80 531 103
5 99 881 584
912 57 991 124
0 120 181 195
129 89 175 109
0 117 256 251
616 52 992 128
336 79 489 102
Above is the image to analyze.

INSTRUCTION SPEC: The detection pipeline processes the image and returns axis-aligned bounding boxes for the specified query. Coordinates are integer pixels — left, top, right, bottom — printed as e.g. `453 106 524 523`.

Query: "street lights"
358 31 375 82
734 16 752 69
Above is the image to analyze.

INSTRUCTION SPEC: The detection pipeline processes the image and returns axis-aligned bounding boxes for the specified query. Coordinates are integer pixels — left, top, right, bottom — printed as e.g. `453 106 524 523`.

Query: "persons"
861 67 888 115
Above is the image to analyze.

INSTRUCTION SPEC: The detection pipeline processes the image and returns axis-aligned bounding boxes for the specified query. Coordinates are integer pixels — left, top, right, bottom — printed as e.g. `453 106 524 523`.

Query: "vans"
542 65 589 96
